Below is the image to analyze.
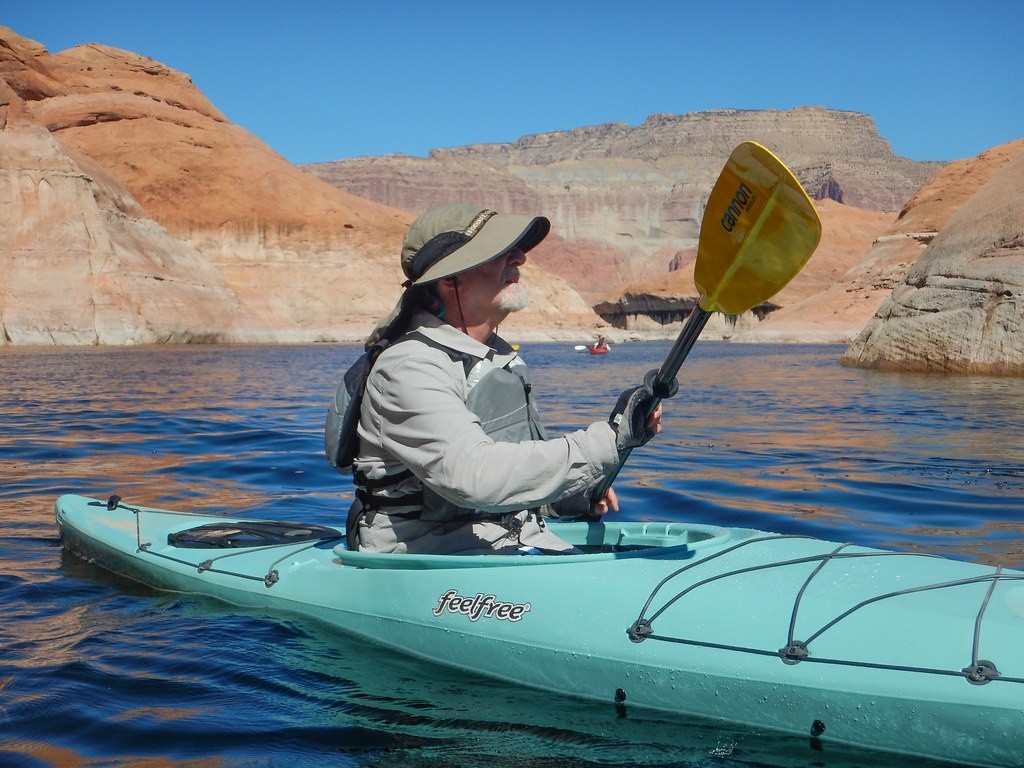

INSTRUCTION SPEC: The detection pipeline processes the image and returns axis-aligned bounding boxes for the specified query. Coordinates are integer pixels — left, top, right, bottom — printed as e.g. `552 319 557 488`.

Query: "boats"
588 346 607 354
54 490 1024 768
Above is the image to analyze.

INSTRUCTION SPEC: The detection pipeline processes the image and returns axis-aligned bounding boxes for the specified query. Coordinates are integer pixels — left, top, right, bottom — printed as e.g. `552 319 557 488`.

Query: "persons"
594 335 611 350
346 204 664 555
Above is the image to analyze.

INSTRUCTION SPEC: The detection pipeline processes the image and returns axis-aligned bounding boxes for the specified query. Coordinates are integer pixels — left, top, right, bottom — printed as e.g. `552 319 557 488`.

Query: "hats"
401 203 551 285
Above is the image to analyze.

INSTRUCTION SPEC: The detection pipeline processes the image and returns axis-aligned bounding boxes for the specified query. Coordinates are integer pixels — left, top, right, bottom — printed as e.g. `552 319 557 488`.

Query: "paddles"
583 142 823 516
574 342 612 351
